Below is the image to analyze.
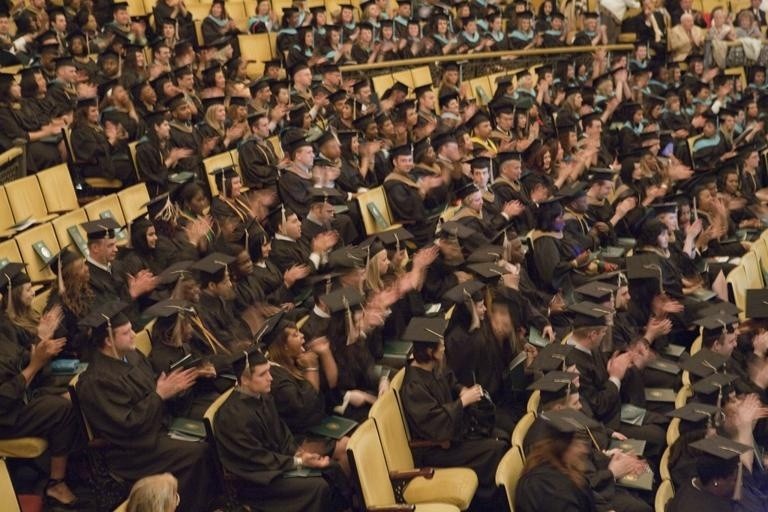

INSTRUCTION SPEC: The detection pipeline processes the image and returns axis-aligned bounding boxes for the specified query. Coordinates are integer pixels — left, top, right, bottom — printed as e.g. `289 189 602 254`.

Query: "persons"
2 2 768 512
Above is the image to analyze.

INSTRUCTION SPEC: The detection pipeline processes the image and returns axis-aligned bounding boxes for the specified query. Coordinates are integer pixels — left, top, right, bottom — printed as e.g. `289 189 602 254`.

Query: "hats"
174 39 191 54
621 103 642 110
432 132 458 151
468 262 510 313
516 98 532 109
338 131 356 142
321 64 340 74
77 98 97 107
667 61 680 69
688 436 752 500
526 371 579 406
143 109 172 122
438 220 475 240
700 170 717 182
702 110 725 130
80 218 121 266
155 260 193 301
734 140 758 152
246 79 269 93
431 11 454 34
533 196 564 216
438 61 465 85
646 93 666 106
450 0 469 9
175 64 192 75
282 8 298 14
634 208 656 236
713 75 740 82
254 312 288 355
591 167 616 180
108 2 128 9
0 74 15 82
412 83 434 97
51 57 76 66
592 72 615 83
119 212 148 249
76 5 89 27
631 69 652 74
718 154 742 184
583 12 599 18
287 60 308 76
461 157 494 186
696 300 743 316
556 120 574 129
124 45 148 69
323 25 342 32
388 144 410 155
247 112 266 124
578 112 604 119
726 101 745 110
230 97 250 106
661 88 677 98
98 49 118 56
492 224 513 260
151 73 169 85
392 80 409 94
439 95 457 108
465 111 489 130
455 124 468 137
666 401 719 437
66 32 88 41
114 29 131 43
164 93 185 107
1 263 31 318
691 80 710 97
309 188 342 221
195 252 238 301
647 202 677 216
207 35 234 50
549 11 564 20
203 96 225 104
588 270 628 287
141 299 195 347
405 19 422 24
355 22 373 29
377 228 414 265
663 192 697 222
262 61 282 67
680 175 701 191
350 79 369 88
97 79 118 95
525 344 575 374
163 17 180 40
455 183 479 199
393 98 417 110
536 409 601 452
40 243 82 296
131 16 150 23
351 112 374 127
226 343 266 374
297 25 312 31
517 11 533 19
400 317 447 378
682 53 706 63
271 79 294 90
632 145 655 156
310 6 326 12
568 301 615 351
78 302 128 359
689 373 739 406
460 15 478 26
468 245 506 265
148 37 165 49
627 254 663 295
556 180 589 203
677 83 687 104
563 86 583 94
325 89 347 102
226 216 260 252
284 134 311 155
359 236 383 279
717 107 738 118
320 285 363 346
209 165 236 201
380 19 393 27
170 171 197 205
535 64 552 73
488 11 503 24
575 281 620 309
496 152 520 164
282 104 307 115
45 6 65 15
130 80 149 91
337 5 355 8
202 62 223 76
262 201 294 237
514 69 529 77
225 54 241 68
617 150 650 170
313 132 334 147
345 97 362 120
443 279 486 333
681 348 727 377
521 139 541 161
746 289 768 318
139 192 177 223
34 43 59 54
16 66 41 76
360 1 376 11
395 0 412 6
33 30 57 43
639 132 660 143
692 313 739 334
493 102 514 113
413 136 432 149
494 75 513 86
308 272 346 296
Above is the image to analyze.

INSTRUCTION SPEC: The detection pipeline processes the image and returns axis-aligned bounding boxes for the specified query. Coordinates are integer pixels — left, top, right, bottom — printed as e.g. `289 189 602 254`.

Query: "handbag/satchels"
462 388 496 440
52 358 80 371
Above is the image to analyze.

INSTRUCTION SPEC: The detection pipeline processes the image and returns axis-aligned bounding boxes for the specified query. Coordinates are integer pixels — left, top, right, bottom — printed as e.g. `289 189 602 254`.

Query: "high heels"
43 478 89 507
